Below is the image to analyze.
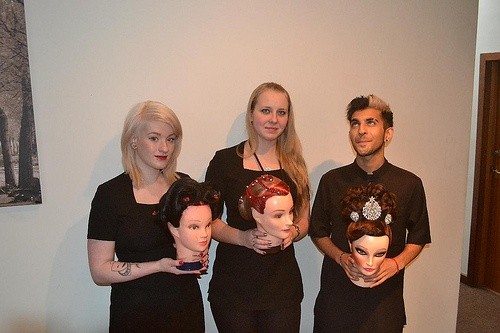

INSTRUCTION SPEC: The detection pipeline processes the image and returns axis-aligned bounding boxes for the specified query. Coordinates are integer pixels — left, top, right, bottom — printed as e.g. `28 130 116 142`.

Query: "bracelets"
391 257 399 274
339 252 346 266
292 224 300 241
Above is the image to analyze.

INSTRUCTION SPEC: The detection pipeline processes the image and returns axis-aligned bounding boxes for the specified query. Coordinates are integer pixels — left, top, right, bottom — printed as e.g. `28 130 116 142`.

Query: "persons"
87 100 209 333
204 82 310 333
238 174 293 247
158 179 224 272
335 181 398 288
308 94 431 333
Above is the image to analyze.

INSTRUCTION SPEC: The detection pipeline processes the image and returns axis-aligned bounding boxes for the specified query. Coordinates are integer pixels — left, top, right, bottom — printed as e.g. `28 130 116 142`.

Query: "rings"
252 238 255 243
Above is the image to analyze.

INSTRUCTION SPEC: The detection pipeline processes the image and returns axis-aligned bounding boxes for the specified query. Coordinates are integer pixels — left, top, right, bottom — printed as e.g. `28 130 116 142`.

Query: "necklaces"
247 139 286 182
